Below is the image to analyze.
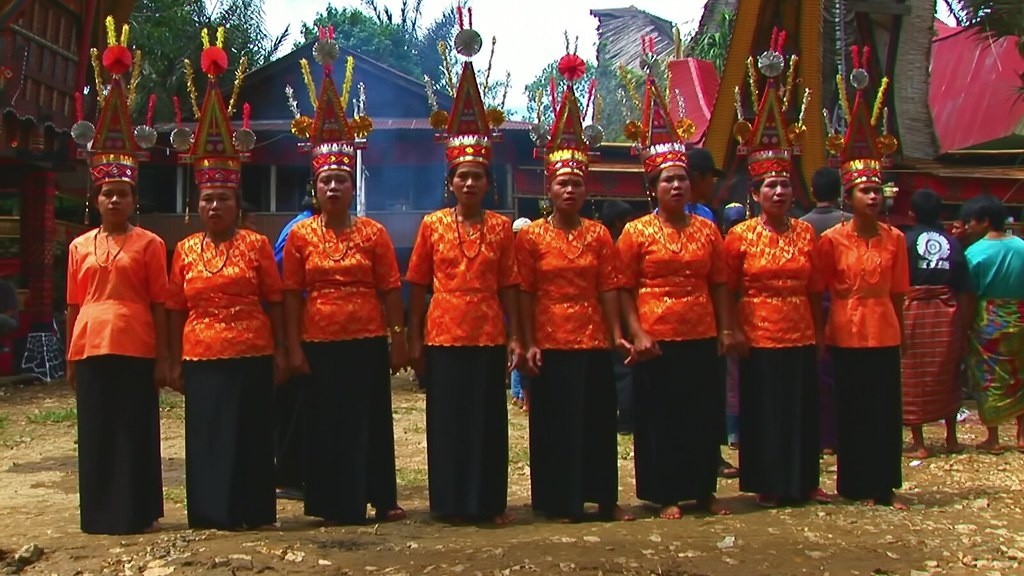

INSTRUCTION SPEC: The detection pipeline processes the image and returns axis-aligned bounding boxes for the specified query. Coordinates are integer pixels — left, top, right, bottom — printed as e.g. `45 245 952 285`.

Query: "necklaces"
853 232 882 283
93 226 127 267
201 230 233 272
654 210 684 252
548 212 586 259
318 214 353 261
759 214 793 256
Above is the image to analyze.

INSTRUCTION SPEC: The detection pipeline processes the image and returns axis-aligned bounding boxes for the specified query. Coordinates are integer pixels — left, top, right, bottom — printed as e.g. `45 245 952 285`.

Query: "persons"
403 162 522 529
273 204 321 495
454 205 485 259
613 166 732 521
513 174 633 523
67 180 167 531
812 182 912 510
279 169 407 526
169 185 279 529
597 169 1023 457
722 176 835 511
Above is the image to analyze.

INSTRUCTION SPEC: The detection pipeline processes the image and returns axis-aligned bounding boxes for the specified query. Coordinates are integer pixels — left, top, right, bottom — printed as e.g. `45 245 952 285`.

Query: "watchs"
390 323 405 334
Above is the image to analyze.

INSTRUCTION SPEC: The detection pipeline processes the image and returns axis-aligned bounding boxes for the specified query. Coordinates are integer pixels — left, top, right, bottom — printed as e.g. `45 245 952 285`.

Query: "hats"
685 149 724 177
284 25 373 177
71 15 157 185
733 26 812 182
723 203 745 222
529 29 605 185
619 35 695 187
170 27 256 189
822 45 897 191
423 5 505 169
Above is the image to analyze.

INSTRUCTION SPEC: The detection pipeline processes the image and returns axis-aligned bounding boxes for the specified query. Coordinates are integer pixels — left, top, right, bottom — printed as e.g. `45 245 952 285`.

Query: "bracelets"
510 335 521 340
